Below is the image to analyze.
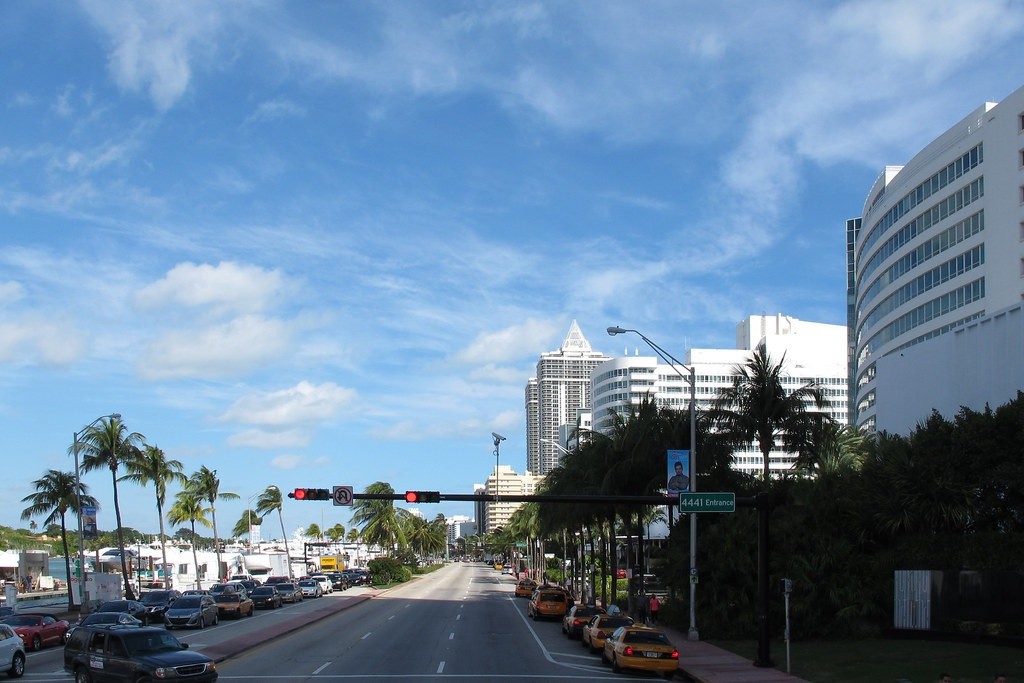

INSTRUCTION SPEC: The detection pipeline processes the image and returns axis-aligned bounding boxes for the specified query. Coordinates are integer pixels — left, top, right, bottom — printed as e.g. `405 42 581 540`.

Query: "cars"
484 558 513 575
417 558 443 568
533 584 574 612
601 622 679 680
581 604 636 655
606 565 669 605
514 578 538 598
561 604 608 640
527 588 571 622
90 600 151 626
65 612 143 644
216 592 255 620
0 624 26 678
209 567 375 610
181 589 213 596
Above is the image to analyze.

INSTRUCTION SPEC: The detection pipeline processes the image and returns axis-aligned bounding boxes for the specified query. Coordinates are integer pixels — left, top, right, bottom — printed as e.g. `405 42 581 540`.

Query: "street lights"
607 325 700 642
73 413 122 619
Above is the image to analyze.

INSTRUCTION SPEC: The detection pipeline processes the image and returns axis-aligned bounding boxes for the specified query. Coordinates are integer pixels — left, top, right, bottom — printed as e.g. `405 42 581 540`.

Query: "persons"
637 592 648 624
650 594 660 624
20 573 33 593
566 577 571 591
667 462 689 490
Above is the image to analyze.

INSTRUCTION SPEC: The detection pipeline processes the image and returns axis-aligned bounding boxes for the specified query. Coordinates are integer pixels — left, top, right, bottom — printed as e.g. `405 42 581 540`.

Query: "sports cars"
0 612 70 651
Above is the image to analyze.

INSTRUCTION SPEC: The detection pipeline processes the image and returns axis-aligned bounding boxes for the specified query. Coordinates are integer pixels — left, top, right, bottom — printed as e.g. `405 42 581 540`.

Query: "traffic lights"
295 488 329 500
406 490 441 504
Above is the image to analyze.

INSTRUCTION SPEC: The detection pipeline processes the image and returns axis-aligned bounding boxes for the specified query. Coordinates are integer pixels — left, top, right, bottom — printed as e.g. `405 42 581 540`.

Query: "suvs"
163 595 218 630
136 589 182 623
63 623 218 683
